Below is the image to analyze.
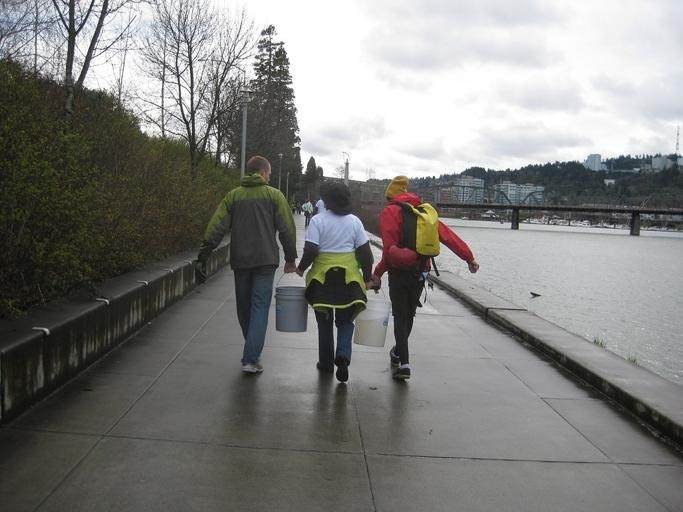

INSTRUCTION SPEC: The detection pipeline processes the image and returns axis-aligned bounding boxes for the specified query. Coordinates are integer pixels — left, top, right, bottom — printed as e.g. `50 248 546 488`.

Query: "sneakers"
317 361 334 373
335 355 349 382
392 367 411 380
241 363 264 373
390 346 400 366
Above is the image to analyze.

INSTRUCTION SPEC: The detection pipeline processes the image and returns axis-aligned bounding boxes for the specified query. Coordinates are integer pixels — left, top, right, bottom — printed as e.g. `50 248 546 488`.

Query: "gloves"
195 262 207 283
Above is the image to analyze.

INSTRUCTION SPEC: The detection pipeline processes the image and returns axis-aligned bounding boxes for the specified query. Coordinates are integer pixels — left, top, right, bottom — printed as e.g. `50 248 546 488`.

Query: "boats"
521 214 679 232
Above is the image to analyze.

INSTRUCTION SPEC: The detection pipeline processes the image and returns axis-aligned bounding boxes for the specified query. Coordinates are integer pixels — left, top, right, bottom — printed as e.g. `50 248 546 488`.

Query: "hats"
385 176 409 199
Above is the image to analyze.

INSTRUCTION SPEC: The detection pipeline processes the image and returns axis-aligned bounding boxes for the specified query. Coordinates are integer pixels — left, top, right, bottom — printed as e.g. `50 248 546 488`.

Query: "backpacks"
393 201 441 257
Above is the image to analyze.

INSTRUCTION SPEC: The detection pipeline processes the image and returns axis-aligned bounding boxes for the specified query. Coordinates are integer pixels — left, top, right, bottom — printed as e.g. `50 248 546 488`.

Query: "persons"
297 199 326 228
373 175 480 380
194 154 298 375
297 179 375 382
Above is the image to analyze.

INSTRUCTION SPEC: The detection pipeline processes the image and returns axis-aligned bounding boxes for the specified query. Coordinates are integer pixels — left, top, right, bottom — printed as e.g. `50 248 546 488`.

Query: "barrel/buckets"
354 288 391 348
276 270 310 333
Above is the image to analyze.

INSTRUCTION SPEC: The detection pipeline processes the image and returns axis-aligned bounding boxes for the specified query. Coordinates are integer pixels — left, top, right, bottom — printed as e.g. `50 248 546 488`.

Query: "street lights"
285 171 290 199
195 57 250 89
278 152 283 191
239 80 252 178
